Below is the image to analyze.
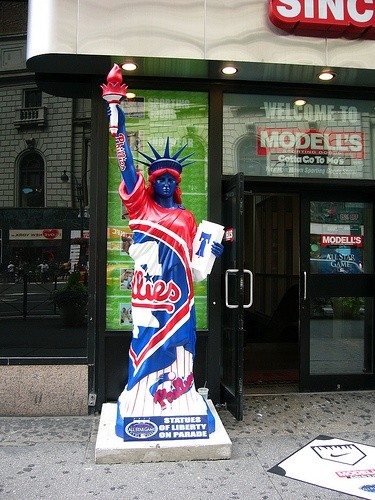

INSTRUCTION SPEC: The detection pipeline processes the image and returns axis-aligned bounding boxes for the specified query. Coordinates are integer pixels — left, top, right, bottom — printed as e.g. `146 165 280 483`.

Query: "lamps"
60 170 69 184
26 137 35 146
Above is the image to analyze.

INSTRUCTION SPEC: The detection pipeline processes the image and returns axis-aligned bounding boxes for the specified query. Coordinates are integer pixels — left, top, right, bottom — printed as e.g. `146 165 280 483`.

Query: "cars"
322 305 334 317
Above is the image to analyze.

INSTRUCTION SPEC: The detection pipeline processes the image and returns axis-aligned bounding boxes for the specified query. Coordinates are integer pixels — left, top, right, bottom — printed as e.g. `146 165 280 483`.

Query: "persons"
9 256 87 284
106 104 224 438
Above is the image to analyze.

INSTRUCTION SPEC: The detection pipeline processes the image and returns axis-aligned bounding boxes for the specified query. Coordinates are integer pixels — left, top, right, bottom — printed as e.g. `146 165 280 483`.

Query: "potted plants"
52 271 88 328
